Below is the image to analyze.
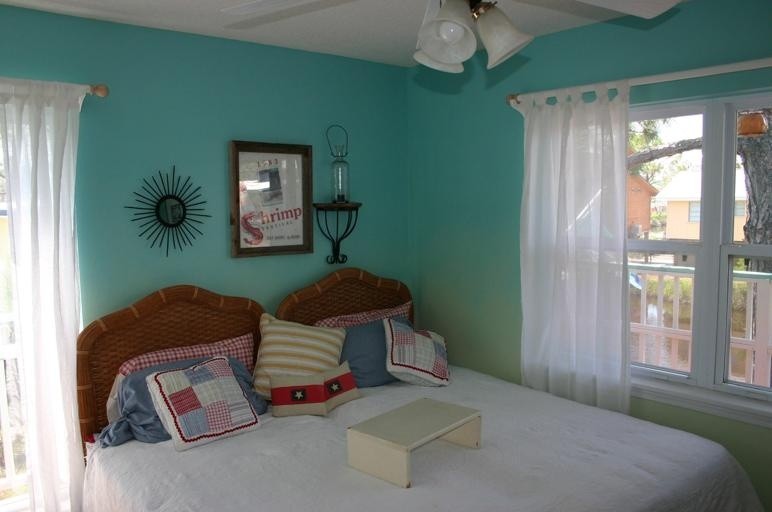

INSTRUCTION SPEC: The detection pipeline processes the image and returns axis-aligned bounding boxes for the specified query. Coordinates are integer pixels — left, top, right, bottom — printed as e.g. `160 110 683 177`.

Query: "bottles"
330 143 350 203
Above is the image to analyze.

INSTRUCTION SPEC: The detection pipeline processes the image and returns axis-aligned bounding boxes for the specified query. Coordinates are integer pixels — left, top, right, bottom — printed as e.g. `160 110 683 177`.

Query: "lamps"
411 1 537 75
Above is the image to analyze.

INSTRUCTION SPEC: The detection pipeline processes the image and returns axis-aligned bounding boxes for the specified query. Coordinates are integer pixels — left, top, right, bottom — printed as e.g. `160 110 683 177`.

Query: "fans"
217 0 683 29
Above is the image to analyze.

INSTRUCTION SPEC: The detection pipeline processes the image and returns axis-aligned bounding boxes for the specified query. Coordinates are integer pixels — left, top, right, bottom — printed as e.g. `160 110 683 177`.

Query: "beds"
75 268 737 512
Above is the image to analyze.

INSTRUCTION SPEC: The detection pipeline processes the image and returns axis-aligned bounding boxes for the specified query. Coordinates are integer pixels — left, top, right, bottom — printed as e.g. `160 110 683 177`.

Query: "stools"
346 396 484 490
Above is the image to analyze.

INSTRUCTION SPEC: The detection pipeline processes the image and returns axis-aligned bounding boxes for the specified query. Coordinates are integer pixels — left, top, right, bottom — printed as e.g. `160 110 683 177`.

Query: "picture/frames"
226 139 315 259
